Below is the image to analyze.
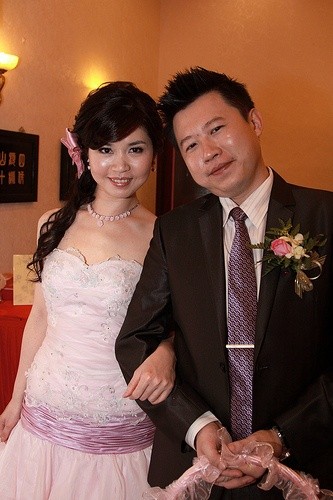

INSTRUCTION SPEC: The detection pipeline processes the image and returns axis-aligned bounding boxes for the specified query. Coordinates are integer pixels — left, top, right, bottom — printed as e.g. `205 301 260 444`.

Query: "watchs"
270 423 292 463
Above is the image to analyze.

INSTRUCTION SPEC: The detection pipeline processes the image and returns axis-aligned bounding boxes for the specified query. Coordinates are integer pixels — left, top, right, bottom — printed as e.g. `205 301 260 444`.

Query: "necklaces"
86 201 142 228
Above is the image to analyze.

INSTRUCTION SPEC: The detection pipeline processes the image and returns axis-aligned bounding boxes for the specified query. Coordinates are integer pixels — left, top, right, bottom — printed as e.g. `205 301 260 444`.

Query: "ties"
225 207 257 442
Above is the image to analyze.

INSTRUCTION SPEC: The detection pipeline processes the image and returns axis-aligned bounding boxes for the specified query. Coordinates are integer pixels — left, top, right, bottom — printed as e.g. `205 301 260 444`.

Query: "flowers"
59 128 85 180
247 218 330 298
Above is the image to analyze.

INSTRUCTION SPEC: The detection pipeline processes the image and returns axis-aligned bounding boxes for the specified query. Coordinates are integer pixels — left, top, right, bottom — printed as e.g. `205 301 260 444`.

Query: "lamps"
0 52 19 102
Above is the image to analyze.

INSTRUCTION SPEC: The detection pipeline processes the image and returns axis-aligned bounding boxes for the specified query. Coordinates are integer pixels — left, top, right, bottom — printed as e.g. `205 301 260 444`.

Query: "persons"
0 80 178 499
115 67 333 499
